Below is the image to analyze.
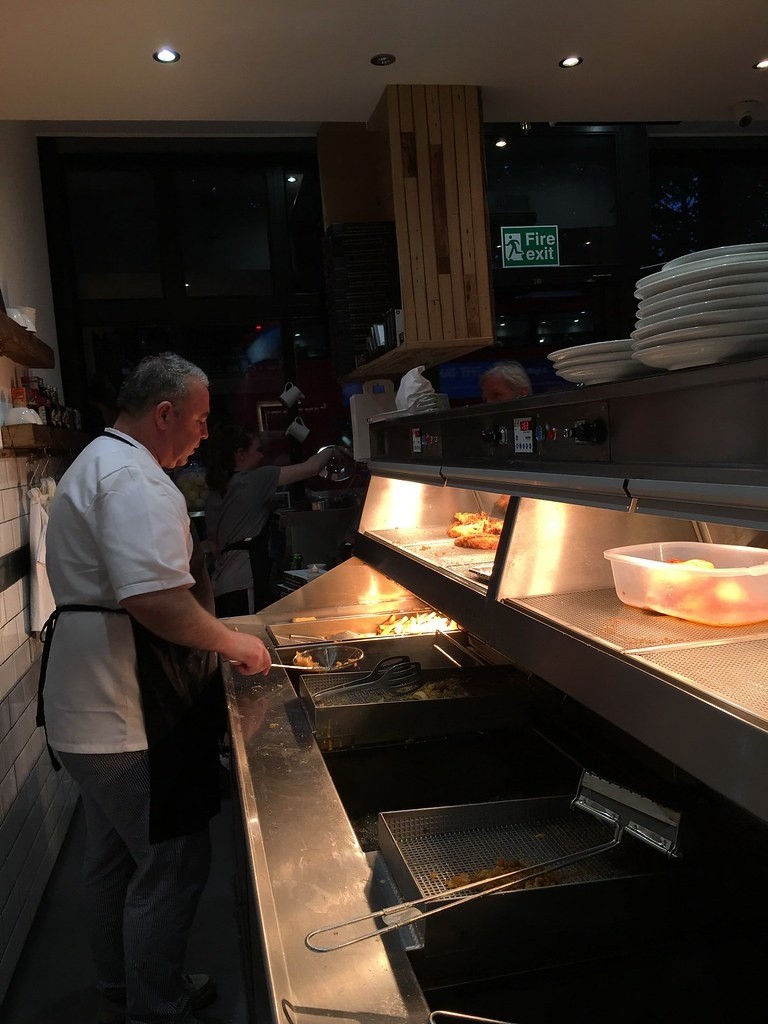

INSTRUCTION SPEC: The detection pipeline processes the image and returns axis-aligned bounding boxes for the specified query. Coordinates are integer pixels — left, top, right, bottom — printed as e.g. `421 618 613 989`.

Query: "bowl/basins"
4 407 44 425
603 541 768 627
6 308 28 329
21 313 37 333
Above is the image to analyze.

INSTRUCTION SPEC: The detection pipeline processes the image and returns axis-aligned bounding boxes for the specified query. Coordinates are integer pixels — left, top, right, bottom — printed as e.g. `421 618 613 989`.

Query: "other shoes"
96 974 217 1024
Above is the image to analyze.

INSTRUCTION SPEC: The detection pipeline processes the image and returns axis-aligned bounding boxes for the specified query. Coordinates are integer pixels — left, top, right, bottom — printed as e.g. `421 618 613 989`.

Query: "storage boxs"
604 541 768 627
12 387 50 409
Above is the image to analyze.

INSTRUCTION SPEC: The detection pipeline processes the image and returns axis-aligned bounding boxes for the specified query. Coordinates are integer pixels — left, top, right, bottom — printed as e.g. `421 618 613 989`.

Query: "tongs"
312 656 423 701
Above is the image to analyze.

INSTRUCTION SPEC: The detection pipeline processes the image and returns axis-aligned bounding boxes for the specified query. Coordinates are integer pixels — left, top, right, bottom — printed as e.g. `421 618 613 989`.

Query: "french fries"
292 616 316 623
375 612 457 635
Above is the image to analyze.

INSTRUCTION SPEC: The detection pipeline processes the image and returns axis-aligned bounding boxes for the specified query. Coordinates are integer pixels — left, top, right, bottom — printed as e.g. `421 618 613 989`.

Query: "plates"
547 244 768 385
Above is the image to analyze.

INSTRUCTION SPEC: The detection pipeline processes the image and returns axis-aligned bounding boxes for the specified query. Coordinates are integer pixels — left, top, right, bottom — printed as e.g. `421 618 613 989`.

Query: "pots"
304 487 362 511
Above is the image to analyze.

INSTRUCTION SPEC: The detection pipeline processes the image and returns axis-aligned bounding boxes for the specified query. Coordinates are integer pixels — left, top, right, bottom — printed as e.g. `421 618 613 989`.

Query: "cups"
279 381 301 409
286 417 310 442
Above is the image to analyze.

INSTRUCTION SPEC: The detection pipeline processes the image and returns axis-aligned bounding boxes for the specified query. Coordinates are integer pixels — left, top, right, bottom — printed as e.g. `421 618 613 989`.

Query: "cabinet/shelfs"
345 82 494 379
0 310 75 458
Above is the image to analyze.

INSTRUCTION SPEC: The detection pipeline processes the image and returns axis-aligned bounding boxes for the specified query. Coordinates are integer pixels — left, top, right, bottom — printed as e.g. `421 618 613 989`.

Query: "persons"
36 351 272 1024
205 424 336 619
480 361 534 404
